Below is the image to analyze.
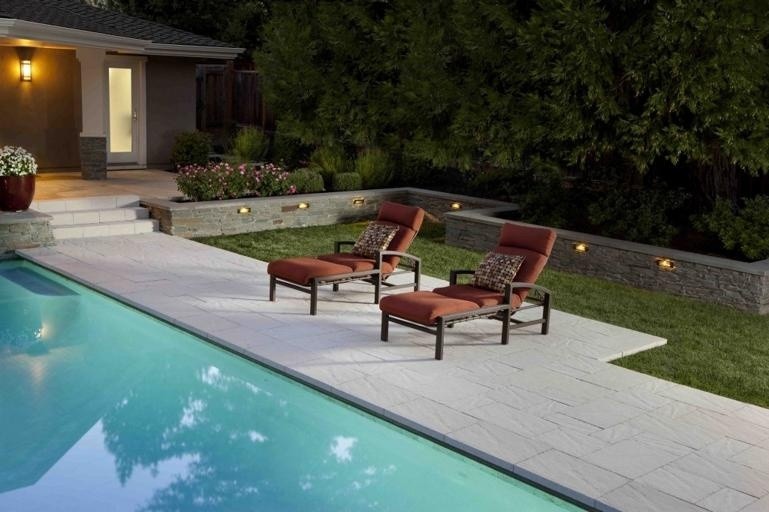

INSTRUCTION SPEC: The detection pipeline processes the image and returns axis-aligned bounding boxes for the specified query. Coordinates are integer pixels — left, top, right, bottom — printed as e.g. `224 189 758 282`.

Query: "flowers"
0 144 37 177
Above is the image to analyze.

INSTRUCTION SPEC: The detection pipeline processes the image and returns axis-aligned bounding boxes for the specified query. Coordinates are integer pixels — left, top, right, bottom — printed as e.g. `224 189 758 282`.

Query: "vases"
0 173 36 212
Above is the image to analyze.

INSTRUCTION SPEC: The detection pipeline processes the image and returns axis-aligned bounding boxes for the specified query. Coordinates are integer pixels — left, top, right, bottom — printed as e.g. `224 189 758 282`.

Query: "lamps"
17 46 35 82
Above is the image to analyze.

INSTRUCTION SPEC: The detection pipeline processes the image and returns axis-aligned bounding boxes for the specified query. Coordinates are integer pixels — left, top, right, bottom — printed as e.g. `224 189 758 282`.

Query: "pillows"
353 222 397 259
471 253 524 290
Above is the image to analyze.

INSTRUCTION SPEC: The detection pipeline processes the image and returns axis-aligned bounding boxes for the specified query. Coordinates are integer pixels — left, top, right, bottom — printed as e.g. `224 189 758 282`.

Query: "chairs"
267 201 427 317
379 223 558 358
